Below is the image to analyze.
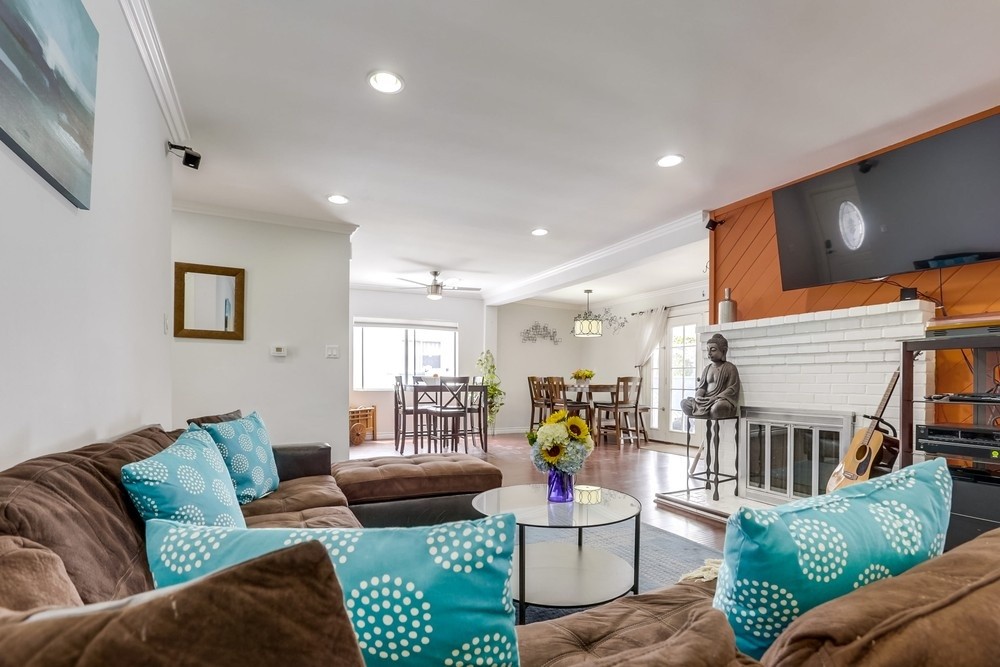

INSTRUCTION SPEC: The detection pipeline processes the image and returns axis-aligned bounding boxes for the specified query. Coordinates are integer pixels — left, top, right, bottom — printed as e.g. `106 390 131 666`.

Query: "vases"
575 376 591 387
546 460 575 503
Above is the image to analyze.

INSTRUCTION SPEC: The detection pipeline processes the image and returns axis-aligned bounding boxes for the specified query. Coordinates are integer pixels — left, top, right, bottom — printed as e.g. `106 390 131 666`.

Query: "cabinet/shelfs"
896 332 1000 473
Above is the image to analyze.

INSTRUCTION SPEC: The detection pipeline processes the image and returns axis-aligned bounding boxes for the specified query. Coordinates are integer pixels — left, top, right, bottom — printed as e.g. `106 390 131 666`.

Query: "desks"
391 384 490 455
534 382 644 447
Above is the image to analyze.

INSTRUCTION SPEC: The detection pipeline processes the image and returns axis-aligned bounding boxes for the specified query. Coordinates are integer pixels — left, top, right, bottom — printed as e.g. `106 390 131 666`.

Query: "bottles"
718 287 737 324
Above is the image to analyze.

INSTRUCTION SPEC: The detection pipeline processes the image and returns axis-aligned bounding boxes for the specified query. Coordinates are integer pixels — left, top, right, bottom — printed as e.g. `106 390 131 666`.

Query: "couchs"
0 406 1000 667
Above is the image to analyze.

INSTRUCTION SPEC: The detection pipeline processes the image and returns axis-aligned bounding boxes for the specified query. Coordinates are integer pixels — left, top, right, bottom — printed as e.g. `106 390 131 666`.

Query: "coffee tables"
470 483 644 625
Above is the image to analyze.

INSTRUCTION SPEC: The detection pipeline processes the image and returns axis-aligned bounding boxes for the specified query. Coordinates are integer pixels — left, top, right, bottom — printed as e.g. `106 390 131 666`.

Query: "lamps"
573 289 603 339
426 286 443 301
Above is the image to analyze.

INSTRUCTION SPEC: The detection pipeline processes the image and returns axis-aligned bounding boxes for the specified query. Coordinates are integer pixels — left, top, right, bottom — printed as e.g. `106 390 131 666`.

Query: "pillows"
120 422 247 528
201 410 281 504
145 512 522 667
713 456 955 662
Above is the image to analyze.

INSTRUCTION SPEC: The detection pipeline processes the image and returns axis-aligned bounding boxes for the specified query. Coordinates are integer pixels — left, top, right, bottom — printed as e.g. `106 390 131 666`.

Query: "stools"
686 414 741 501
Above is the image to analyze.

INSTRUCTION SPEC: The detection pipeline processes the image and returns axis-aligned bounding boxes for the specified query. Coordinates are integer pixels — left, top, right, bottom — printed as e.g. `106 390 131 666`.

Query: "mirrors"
174 261 244 342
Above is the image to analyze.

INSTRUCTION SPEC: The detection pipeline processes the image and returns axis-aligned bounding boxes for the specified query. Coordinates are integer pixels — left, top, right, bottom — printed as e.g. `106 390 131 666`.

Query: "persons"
680 334 740 419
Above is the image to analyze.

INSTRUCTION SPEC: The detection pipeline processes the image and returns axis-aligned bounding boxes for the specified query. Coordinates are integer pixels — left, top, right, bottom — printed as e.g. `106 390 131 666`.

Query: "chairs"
526 375 572 436
426 376 471 455
412 375 458 447
542 376 574 423
603 381 645 445
596 376 643 450
436 375 486 451
546 377 593 447
395 375 438 455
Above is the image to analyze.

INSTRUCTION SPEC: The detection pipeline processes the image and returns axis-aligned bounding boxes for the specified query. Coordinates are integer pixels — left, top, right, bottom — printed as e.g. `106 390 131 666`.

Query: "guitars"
825 350 924 495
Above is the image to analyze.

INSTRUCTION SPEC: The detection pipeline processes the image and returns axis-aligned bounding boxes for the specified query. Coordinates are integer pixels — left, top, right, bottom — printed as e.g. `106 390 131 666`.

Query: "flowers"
570 367 596 382
526 409 596 499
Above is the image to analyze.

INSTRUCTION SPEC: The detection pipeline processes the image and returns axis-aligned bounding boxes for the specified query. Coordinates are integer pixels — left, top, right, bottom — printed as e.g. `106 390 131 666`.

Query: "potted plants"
475 348 507 435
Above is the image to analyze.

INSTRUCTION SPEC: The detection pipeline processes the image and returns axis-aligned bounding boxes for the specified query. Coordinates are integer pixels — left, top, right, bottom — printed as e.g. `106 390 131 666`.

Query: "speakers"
183 150 200 171
705 218 719 231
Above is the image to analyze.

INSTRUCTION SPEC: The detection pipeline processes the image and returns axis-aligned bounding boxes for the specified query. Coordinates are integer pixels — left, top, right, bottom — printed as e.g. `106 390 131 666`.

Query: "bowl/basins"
421 376 440 385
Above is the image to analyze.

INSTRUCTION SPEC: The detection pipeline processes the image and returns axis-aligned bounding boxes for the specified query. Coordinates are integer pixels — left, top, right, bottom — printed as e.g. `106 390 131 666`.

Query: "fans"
394 270 481 293
